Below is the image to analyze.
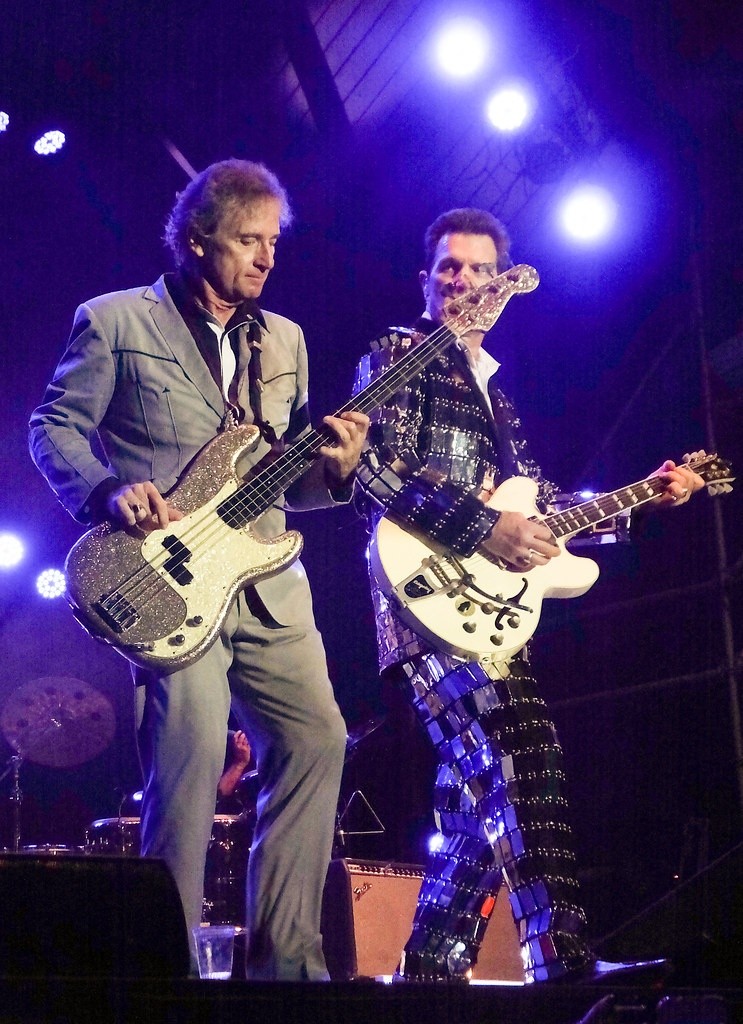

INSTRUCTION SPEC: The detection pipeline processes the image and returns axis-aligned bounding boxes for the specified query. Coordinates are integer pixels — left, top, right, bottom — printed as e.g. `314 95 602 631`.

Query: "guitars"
62 252 538 676
374 445 735 680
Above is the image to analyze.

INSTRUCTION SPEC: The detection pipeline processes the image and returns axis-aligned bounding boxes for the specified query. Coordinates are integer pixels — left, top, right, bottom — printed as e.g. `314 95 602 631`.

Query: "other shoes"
544 957 673 988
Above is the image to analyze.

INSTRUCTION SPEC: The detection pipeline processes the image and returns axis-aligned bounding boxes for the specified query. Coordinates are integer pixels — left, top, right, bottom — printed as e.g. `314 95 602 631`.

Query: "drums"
204 814 251 923
87 815 142 851
18 843 74 867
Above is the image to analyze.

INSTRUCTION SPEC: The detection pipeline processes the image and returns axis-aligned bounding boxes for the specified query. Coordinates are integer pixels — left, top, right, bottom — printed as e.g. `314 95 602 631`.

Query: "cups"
192 925 235 980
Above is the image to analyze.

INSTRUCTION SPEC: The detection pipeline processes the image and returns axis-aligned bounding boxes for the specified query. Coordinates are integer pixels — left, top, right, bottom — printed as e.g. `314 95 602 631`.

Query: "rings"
524 550 533 565
131 504 144 513
683 488 689 497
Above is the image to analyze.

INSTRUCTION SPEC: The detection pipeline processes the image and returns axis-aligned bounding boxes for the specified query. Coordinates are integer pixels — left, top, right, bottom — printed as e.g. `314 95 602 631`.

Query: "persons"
350 208 706 990
29 158 371 984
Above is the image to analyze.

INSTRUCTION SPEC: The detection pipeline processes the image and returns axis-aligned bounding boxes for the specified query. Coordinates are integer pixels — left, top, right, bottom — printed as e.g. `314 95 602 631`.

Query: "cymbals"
347 713 385 747
